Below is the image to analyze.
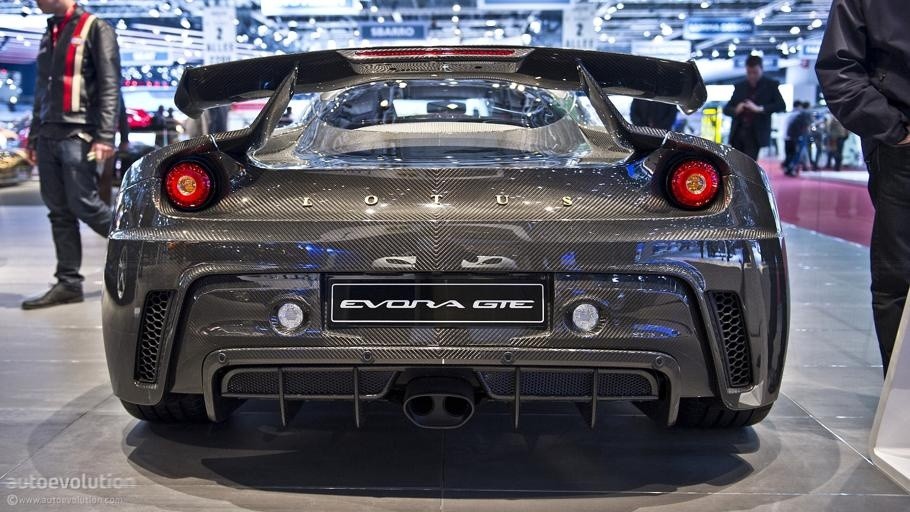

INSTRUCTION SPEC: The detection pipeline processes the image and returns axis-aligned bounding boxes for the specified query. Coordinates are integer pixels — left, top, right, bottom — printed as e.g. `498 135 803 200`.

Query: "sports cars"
97 39 794 438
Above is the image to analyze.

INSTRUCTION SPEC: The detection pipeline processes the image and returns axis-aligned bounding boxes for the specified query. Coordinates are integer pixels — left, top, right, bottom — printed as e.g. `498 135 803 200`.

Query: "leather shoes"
21 279 83 310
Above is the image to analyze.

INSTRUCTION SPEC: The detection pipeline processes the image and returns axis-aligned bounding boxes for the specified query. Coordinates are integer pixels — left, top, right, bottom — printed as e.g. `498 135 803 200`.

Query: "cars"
0 146 36 185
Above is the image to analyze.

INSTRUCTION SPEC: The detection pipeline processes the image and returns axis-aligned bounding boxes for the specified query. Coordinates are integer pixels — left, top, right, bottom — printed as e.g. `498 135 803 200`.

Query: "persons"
152 106 166 150
20 0 129 311
780 99 850 176
165 108 183 145
99 94 128 206
724 56 786 160
813 2 910 381
